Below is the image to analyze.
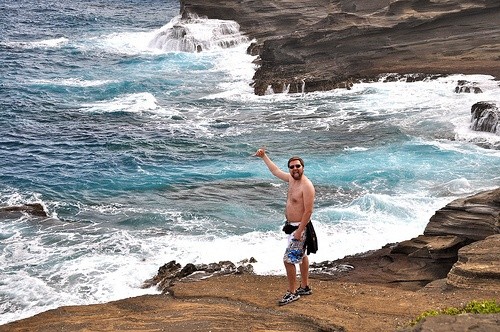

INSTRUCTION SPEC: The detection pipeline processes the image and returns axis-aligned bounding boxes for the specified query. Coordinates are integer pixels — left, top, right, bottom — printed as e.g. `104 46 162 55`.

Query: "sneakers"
294 285 313 297
278 291 300 306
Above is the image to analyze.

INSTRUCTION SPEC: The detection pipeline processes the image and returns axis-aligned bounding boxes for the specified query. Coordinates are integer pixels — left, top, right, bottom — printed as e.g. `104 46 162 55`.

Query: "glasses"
288 164 302 169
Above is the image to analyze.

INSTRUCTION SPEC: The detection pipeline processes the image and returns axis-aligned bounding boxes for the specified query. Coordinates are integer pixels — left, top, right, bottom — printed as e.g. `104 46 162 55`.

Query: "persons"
251 146 316 305
